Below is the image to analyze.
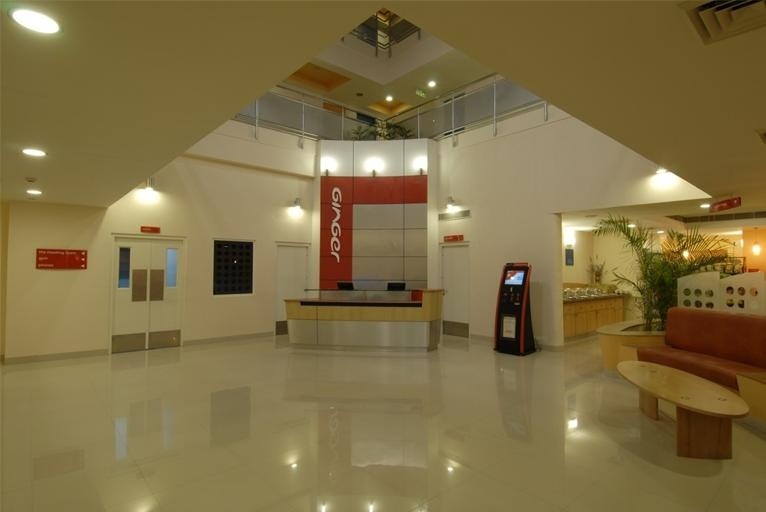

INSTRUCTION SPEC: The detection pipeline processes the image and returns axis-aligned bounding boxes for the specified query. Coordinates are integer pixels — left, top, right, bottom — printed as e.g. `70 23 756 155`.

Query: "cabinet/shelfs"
563 296 623 338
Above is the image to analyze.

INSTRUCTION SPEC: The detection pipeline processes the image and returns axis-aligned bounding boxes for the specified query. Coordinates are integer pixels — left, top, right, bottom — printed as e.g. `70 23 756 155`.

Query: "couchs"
637 306 766 434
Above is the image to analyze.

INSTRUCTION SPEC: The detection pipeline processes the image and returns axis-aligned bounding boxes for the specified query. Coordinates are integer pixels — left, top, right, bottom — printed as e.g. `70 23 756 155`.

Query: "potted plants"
588 210 742 361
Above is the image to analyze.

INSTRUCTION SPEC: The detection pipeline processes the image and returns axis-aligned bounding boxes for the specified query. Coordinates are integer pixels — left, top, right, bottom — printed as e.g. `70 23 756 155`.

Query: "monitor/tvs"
388 282 406 290
503 268 526 287
337 281 354 290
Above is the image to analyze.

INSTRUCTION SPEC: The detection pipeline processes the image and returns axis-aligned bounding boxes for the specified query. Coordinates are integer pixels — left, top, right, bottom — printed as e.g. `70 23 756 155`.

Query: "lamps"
145 177 152 190
295 198 301 207
446 196 454 207
